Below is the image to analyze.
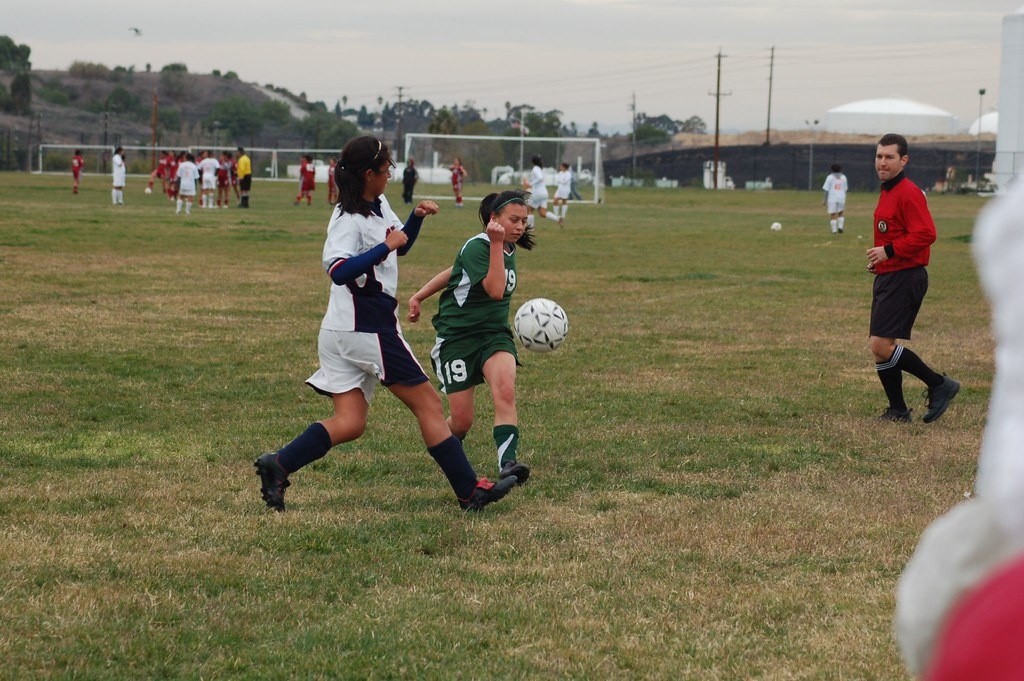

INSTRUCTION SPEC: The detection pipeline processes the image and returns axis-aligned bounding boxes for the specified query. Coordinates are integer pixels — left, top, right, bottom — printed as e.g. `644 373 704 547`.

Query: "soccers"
144 187 152 193
512 297 571 351
770 222 782 231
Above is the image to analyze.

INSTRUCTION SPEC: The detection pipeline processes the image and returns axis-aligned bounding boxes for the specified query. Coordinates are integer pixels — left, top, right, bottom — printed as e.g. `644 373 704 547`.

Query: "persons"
112 147 126 206
149 150 239 208
893 171 1024 681
176 153 199 214
866 134 960 423
402 160 417 204
254 136 514 513
408 191 529 487
569 167 583 200
523 156 563 229
822 165 848 233
294 155 316 205
554 164 571 218
72 150 83 193
449 158 467 208
328 158 338 203
236 147 251 207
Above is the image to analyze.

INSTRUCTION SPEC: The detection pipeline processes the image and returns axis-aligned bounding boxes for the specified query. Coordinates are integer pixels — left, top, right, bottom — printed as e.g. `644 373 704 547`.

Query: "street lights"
975 88 986 187
805 119 818 192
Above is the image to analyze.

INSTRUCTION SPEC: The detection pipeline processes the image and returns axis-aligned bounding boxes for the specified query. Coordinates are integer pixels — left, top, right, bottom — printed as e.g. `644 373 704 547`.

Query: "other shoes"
222 205 227 209
239 204 250 208
831 231 837 235
838 225 843 233
455 203 464 207
558 216 564 227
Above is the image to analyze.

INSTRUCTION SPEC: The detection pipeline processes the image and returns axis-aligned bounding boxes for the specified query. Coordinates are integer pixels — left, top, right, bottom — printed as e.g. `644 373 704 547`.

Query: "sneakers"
502 464 531 484
255 454 292 511
462 474 519 509
923 375 960 423
878 407 913 424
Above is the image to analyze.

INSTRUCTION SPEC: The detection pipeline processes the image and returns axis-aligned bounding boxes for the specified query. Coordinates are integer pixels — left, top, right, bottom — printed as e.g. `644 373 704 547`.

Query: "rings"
871 258 874 261
